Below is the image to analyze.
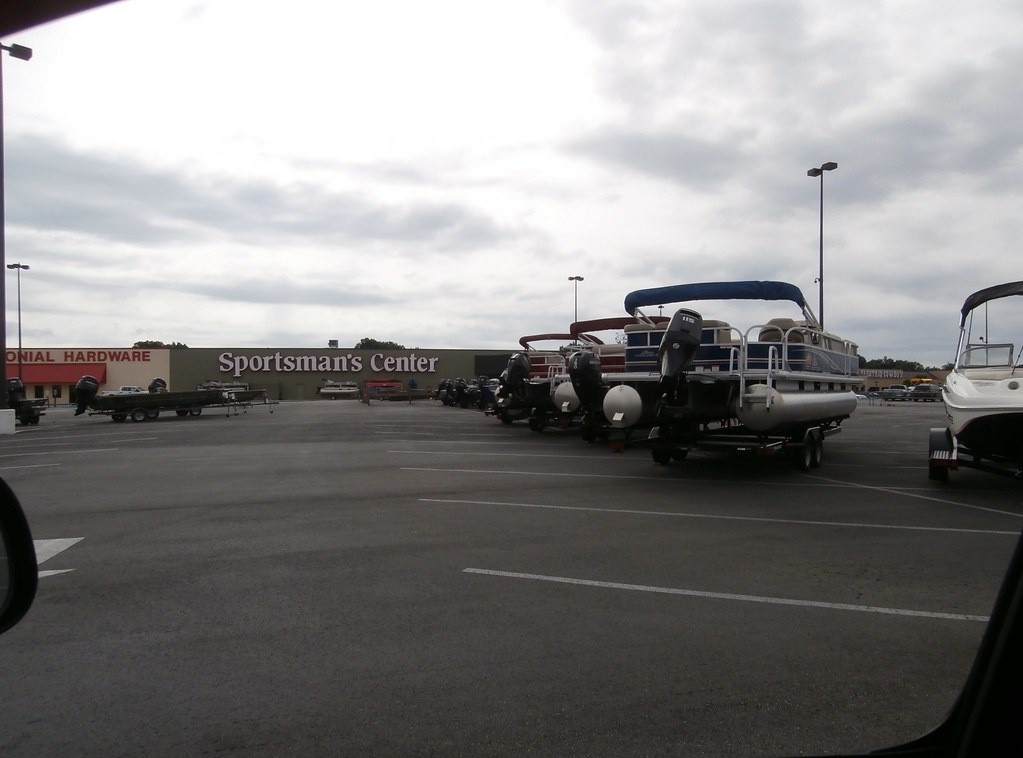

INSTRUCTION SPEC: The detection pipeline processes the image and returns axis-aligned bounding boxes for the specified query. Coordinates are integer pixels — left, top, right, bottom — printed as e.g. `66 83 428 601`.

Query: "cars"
850 390 883 400
911 383 941 402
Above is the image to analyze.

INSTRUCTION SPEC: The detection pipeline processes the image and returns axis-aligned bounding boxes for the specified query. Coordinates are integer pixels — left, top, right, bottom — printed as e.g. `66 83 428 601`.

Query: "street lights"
7 262 30 381
569 275 584 348
807 161 839 338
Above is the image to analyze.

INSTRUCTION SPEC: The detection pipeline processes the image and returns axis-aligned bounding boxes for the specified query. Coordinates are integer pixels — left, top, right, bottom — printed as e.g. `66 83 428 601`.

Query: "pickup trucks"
884 384 911 401
101 385 150 396
